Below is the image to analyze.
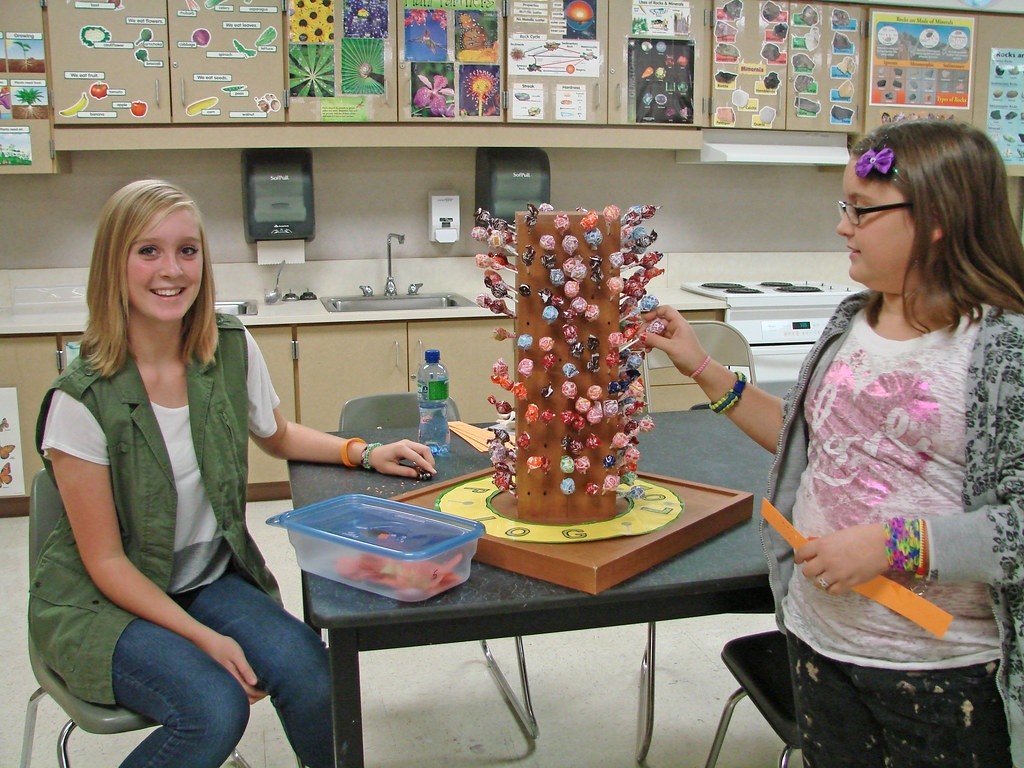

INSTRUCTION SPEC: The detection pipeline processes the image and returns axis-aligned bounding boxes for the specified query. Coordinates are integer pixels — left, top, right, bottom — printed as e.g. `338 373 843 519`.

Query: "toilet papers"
240 149 318 265
475 147 552 236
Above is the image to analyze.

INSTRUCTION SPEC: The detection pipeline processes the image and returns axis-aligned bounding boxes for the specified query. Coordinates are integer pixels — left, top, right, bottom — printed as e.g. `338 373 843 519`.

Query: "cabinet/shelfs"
0 307 729 519
0 0 1024 178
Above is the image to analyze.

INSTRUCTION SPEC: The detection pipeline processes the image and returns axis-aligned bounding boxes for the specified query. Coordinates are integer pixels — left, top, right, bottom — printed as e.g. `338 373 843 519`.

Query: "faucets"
383 233 405 296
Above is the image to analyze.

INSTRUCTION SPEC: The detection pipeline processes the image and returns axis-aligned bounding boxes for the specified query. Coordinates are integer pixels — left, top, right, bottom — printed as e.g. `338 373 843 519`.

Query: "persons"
644 124 1024 768
29 181 437 768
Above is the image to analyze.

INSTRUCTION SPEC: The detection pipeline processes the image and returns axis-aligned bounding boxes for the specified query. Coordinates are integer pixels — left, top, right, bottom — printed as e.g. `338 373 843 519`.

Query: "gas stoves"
682 280 866 345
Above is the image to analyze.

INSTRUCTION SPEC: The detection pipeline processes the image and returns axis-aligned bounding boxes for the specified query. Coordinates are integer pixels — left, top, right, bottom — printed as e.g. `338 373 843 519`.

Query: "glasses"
835 199 912 225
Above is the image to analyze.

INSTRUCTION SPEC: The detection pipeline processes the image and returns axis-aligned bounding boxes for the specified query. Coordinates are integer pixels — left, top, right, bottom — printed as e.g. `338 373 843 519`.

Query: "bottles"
417 350 450 455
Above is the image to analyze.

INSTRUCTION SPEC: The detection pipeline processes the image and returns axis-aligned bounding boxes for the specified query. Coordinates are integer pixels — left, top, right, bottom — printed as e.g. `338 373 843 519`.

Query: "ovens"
723 345 814 399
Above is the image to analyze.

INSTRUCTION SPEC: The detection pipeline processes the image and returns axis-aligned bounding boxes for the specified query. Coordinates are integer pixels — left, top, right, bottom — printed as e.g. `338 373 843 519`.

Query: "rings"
819 577 831 590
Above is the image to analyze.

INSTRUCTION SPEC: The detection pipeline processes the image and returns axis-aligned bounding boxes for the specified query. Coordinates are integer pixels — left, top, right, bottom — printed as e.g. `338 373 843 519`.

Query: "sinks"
214 302 249 316
334 297 458 310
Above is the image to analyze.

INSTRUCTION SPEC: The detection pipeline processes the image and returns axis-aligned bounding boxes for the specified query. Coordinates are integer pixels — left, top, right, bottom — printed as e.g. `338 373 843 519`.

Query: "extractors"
674 129 851 166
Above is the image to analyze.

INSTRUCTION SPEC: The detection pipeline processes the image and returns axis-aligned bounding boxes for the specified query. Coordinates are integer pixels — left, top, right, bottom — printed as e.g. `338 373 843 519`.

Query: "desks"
288 408 777 768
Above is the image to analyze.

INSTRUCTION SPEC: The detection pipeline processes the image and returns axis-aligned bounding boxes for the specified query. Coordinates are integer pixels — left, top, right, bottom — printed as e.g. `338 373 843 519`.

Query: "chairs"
335 389 459 431
705 630 804 768
640 319 757 415
21 466 251 768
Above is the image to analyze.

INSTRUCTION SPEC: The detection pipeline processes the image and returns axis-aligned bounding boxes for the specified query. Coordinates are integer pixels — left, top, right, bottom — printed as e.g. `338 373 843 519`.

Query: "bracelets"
709 371 747 415
342 438 366 468
883 518 927 578
690 356 710 377
359 443 382 469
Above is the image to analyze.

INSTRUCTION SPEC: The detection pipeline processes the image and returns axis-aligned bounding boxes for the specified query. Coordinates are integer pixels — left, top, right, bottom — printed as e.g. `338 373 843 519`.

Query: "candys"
471 204 666 496
